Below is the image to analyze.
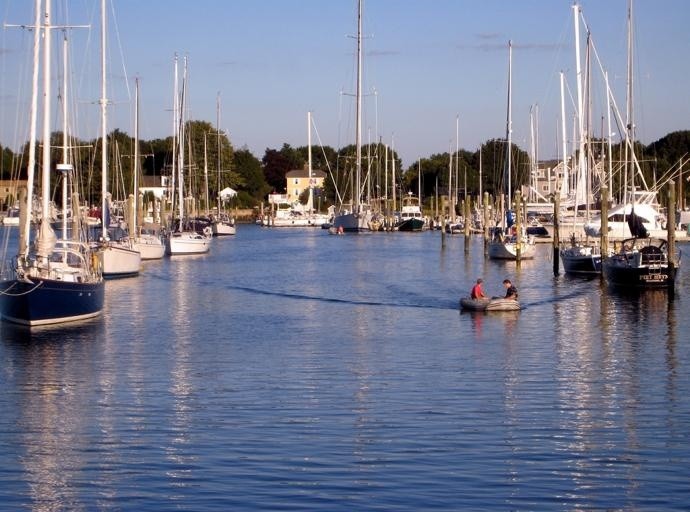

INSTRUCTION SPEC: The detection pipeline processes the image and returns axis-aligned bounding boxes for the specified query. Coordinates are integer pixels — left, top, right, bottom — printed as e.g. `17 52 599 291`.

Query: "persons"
492 278 519 301
471 311 483 344
504 310 520 343
471 277 489 300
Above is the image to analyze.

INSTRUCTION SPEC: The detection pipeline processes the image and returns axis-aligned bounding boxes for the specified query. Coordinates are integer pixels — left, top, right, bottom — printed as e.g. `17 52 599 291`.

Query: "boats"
458 297 522 311
